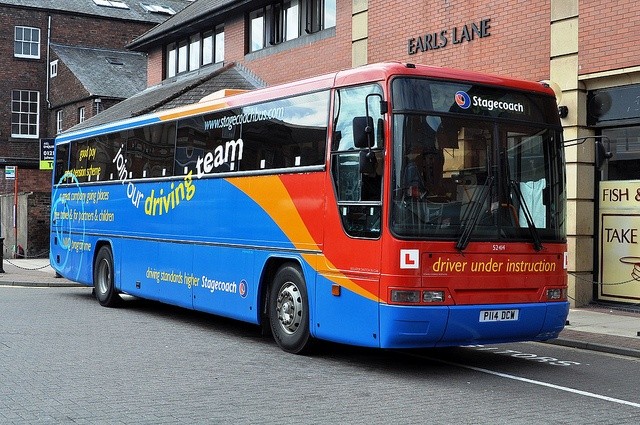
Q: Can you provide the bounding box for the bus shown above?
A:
[49,60,613,354]
